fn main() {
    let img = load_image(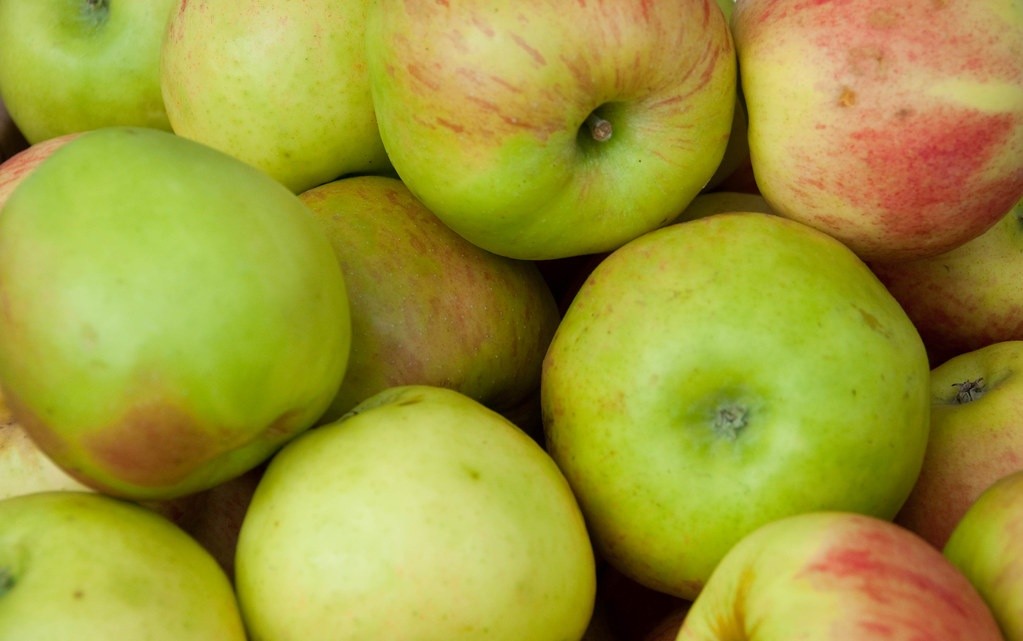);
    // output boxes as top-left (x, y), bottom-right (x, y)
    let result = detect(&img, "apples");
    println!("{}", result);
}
top-left (0, 0), bottom-right (1023, 641)
